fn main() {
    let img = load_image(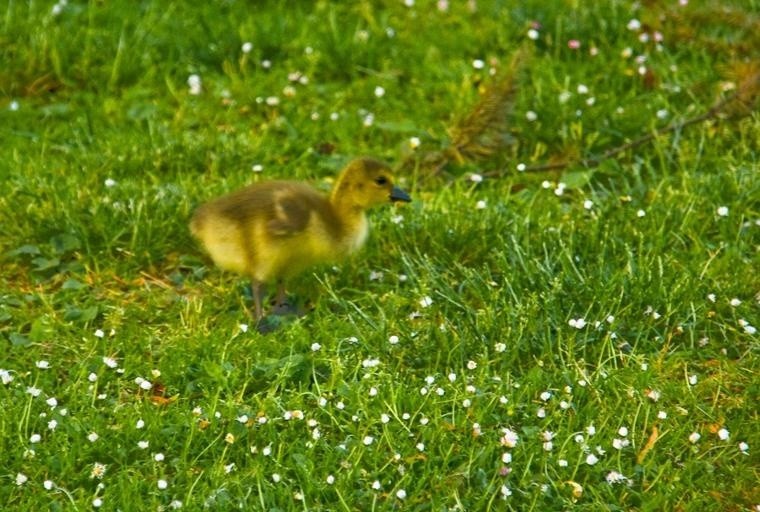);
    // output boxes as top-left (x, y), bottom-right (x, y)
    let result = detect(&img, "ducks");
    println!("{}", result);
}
top-left (186, 157), bottom-right (413, 319)
